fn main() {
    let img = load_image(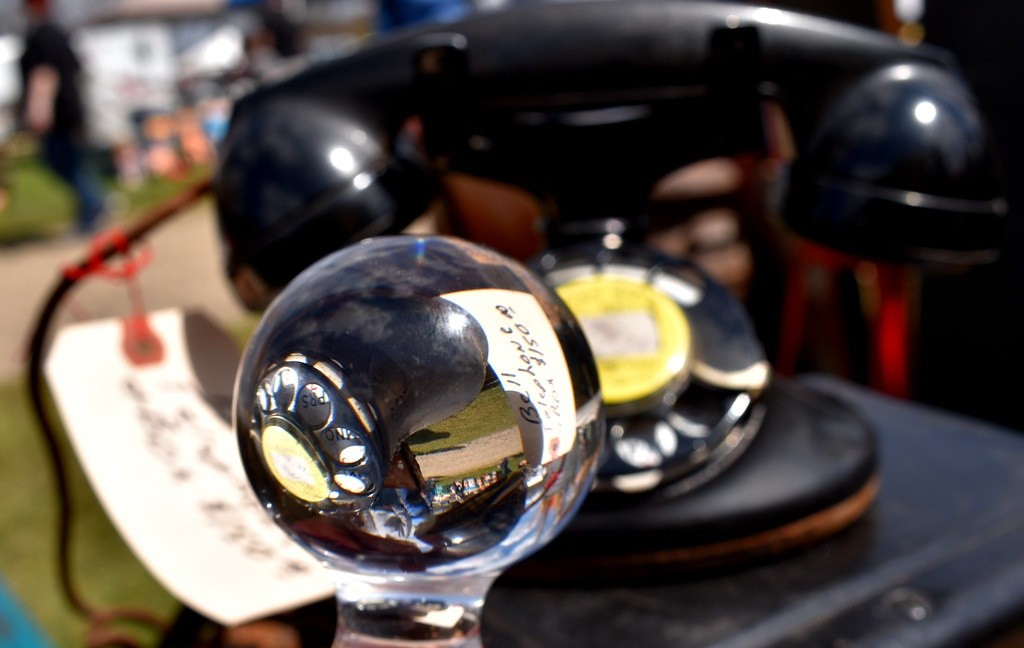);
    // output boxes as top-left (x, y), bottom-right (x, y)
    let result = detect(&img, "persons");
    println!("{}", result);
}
top-left (124, 37), bottom-right (180, 155)
top-left (16, 0), bottom-right (104, 234)
